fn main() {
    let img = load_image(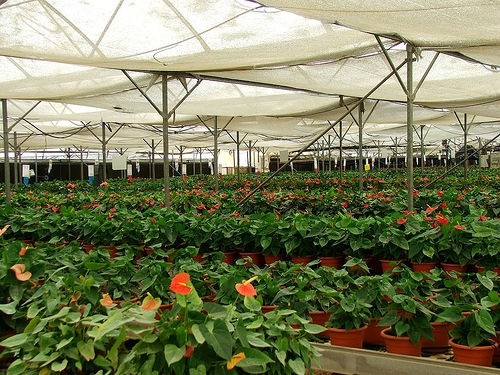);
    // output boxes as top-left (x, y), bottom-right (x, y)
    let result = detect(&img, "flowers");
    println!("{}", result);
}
top-left (0, 165), bottom-right (500, 375)
